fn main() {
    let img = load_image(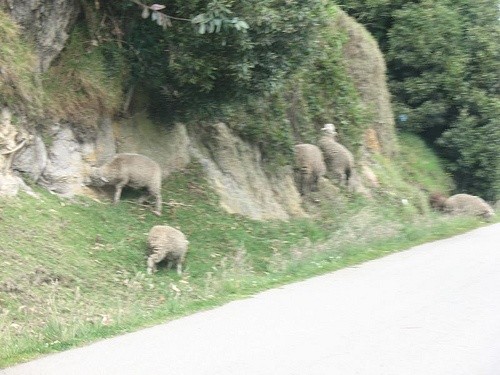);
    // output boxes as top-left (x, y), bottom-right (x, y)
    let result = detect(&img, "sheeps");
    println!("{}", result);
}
top-left (422, 187), bottom-right (496, 220)
top-left (293, 143), bottom-right (327, 196)
top-left (89, 153), bottom-right (162, 214)
top-left (147, 226), bottom-right (190, 276)
top-left (318, 124), bottom-right (354, 187)
